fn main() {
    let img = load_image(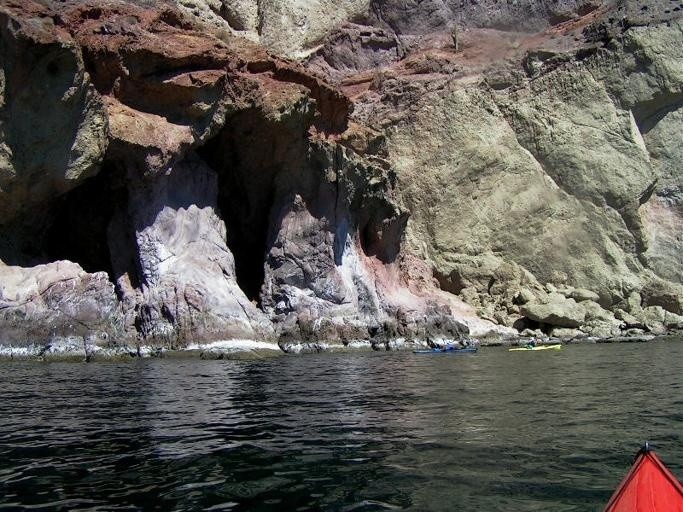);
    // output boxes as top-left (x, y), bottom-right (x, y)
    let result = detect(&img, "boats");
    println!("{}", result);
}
top-left (594, 441), bottom-right (682, 512)
top-left (504, 343), bottom-right (563, 354)
top-left (412, 346), bottom-right (480, 356)
